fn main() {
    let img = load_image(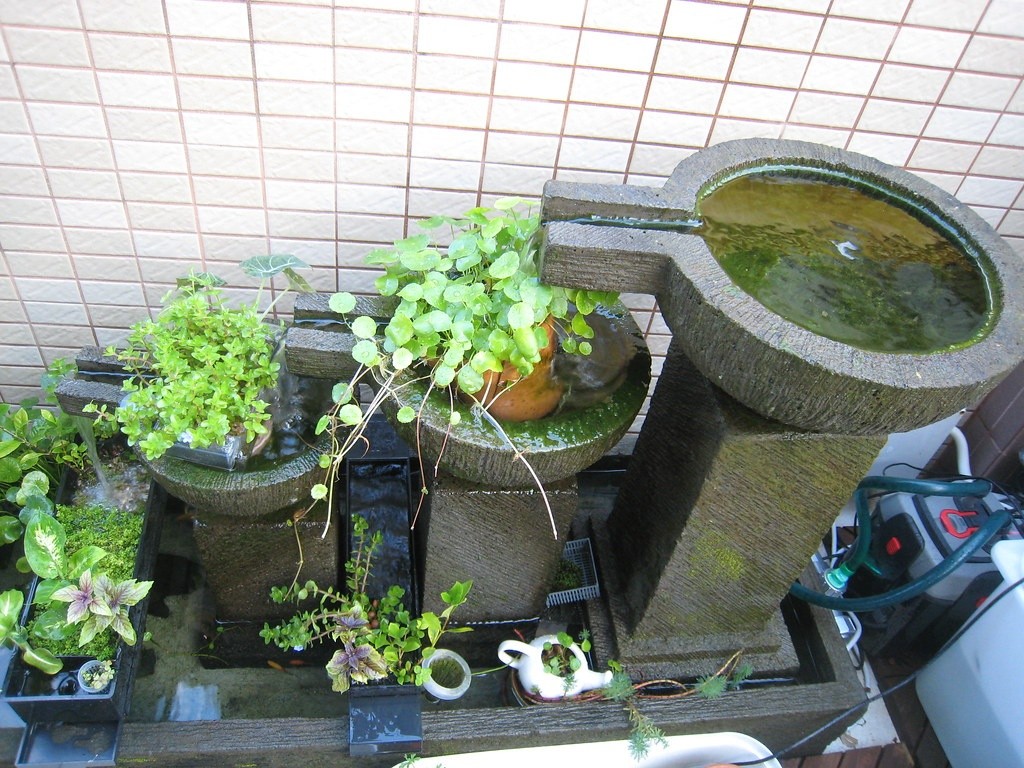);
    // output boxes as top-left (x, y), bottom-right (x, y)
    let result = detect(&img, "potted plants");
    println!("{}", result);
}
top-left (283, 195), bottom-right (621, 600)
top-left (498, 631), bottom-right (613, 699)
top-left (82, 253), bottom-right (315, 469)
